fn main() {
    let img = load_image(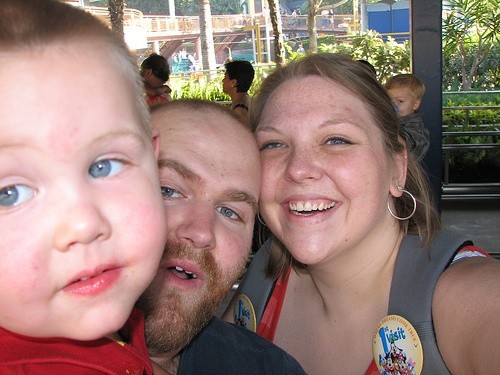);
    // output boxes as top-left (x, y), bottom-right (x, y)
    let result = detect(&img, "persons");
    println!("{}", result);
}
top-left (140, 53), bottom-right (172, 106)
top-left (215, 53), bottom-right (500, 375)
top-left (222, 60), bottom-right (255, 126)
top-left (385, 73), bottom-right (431, 164)
top-left (143, 98), bottom-right (306, 375)
top-left (0, 0), bottom-right (168, 375)
